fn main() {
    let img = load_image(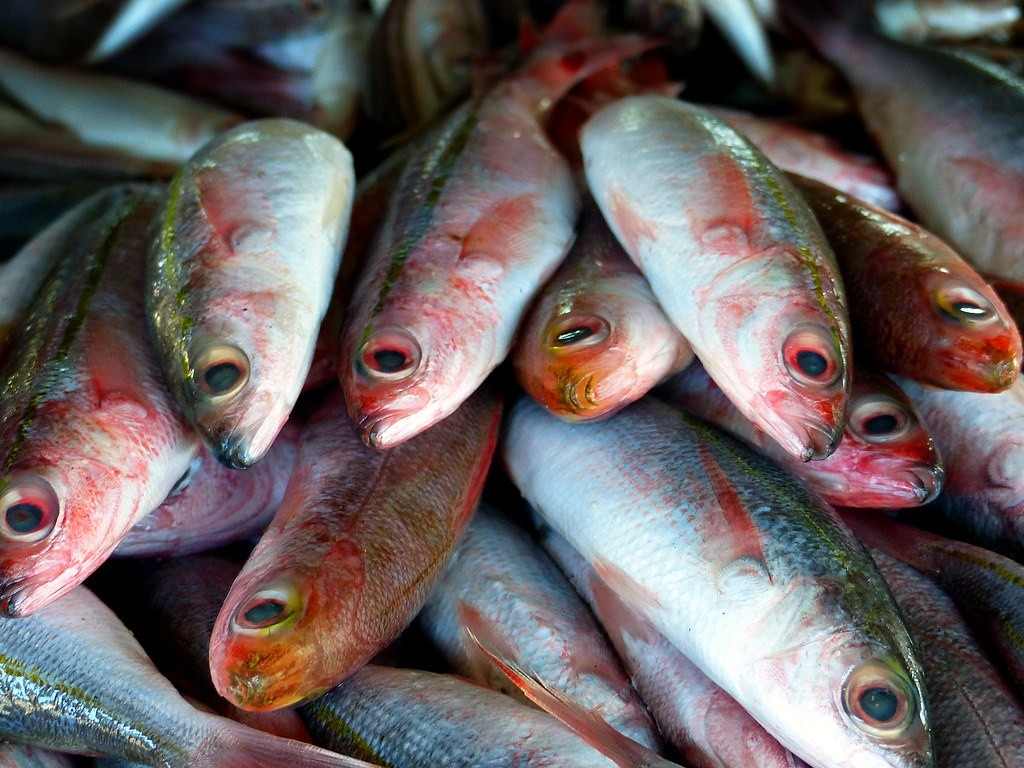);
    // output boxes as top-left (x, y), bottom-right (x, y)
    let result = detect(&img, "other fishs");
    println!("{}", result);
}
top-left (1, 1), bottom-right (1024, 768)
